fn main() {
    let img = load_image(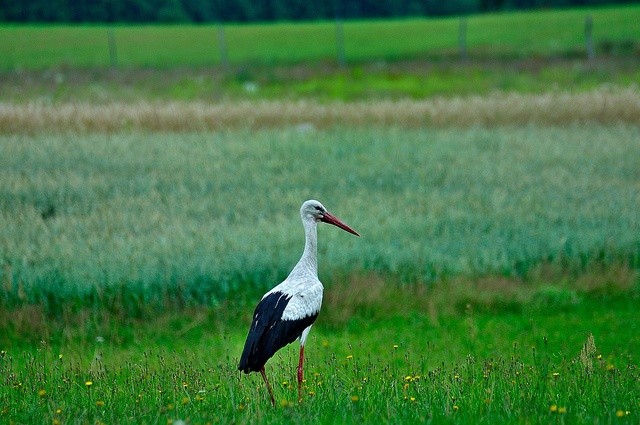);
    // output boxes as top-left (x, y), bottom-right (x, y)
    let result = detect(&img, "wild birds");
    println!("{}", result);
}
top-left (237, 199), bottom-right (361, 412)
